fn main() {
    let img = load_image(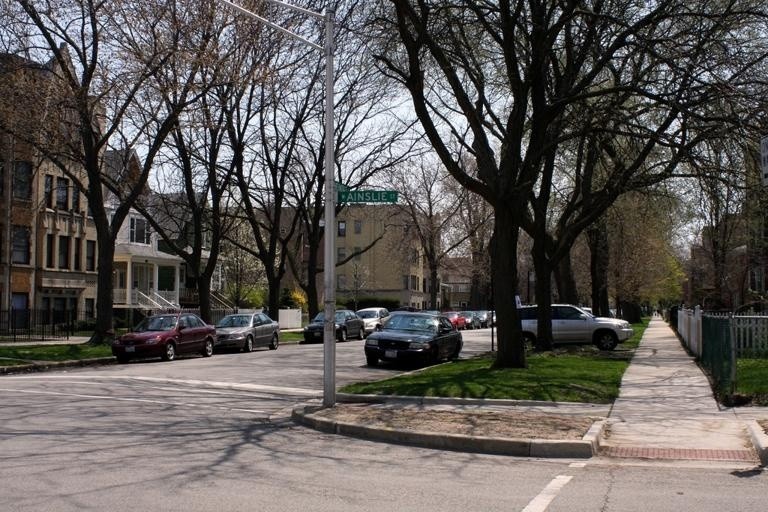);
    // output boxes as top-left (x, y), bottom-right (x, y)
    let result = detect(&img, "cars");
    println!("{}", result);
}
top-left (520, 304), bottom-right (635, 351)
top-left (111, 313), bottom-right (279, 364)
top-left (304, 307), bottom-right (496, 369)
